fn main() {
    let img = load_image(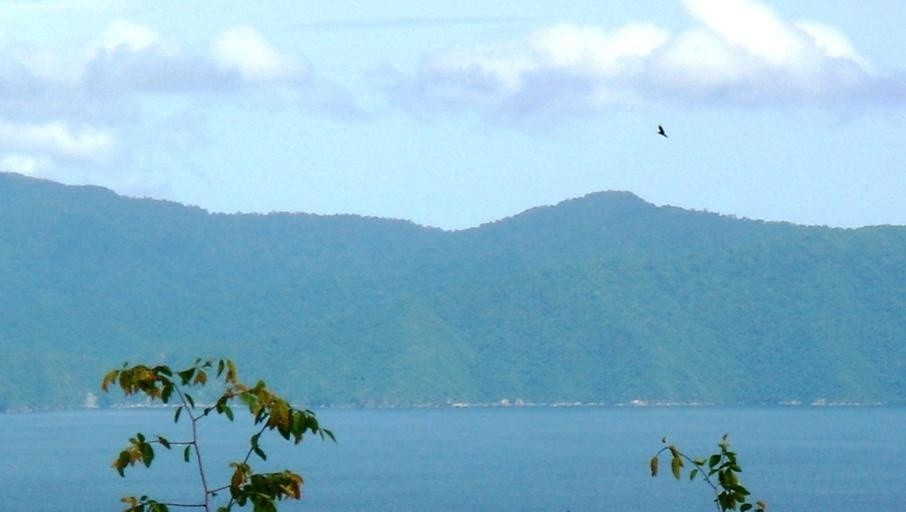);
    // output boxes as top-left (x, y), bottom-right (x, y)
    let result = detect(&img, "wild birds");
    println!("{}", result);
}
top-left (658, 125), bottom-right (668, 138)
